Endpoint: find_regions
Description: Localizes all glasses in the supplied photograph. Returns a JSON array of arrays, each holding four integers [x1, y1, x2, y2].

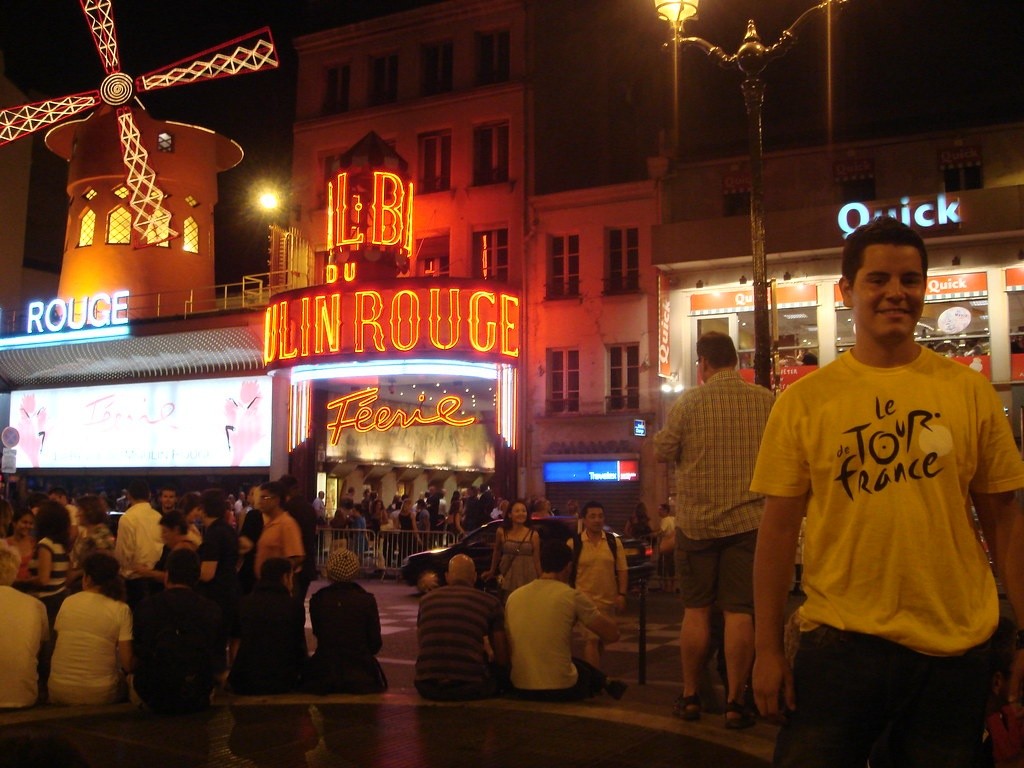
[[260, 495, 276, 501]]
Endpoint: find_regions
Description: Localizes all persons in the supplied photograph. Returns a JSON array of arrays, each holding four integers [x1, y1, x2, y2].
[[567, 502, 628, 668], [625, 502, 677, 592], [504, 542, 630, 700], [1011, 336, 1024, 353], [313, 491, 324, 551], [529, 492, 580, 517], [414, 554, 508, 700], [920, 340, 981, 356], [331, 484, 509, 566], [654, 331, 776, 727], [0, 475, 317, 667], [481, 501, 543, 595], [751, 218, 1024, 768], [800, 343, 817, 364], [0, 549, 388, 711]]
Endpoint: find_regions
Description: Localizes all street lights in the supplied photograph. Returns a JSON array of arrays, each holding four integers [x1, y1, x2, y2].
[[653, 1, 847, 391]]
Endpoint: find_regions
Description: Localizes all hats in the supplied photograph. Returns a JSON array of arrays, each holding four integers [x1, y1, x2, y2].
[[327, 547, 360, 583]]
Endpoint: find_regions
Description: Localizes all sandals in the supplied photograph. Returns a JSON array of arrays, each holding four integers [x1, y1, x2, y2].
[[672, 693, 701, 720], [725, 700, 756, 729]]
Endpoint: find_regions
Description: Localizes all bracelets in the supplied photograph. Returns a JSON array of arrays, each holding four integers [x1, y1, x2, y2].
[[619, 592, 626, 596]]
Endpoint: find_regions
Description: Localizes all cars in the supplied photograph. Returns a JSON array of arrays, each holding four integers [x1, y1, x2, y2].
[[400, 516, 658, 596]]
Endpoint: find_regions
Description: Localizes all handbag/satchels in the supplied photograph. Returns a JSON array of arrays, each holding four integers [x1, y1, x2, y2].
[[485, 575, 504, 595], [380, 510, 394, 531]]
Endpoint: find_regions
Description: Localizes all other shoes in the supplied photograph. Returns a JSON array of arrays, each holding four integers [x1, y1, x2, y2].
[[606, 680, 629, 700]]
